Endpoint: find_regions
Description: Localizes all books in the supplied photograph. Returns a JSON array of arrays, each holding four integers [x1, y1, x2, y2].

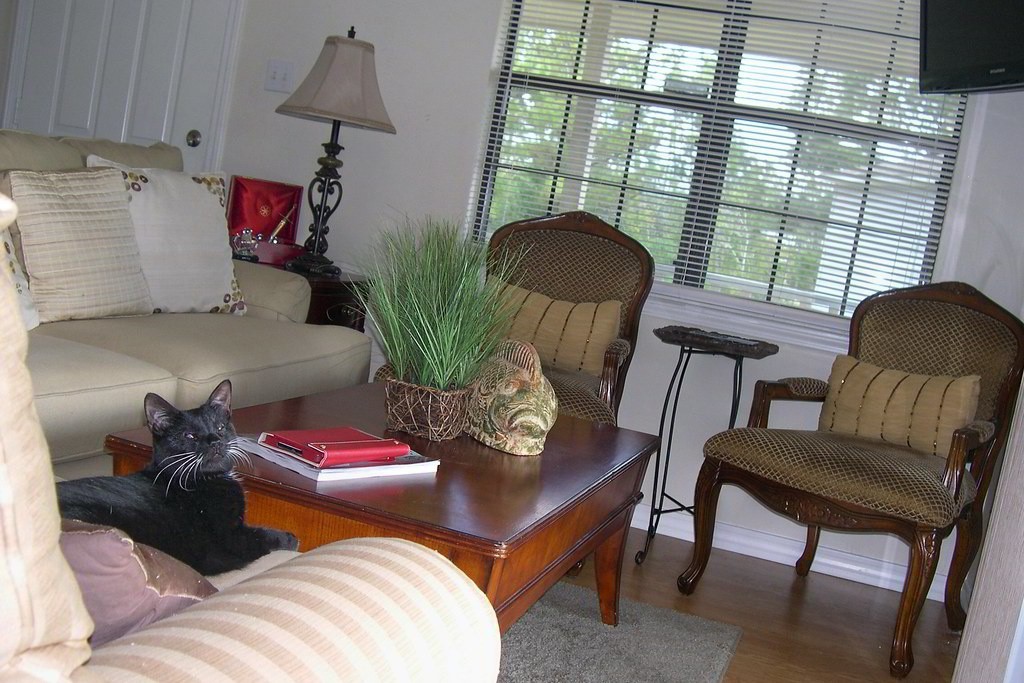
[[235, 426, 441, 482]]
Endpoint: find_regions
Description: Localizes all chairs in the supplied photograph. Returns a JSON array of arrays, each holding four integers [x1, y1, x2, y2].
[[677, 280, 1024, 678], [461, 210, 656, 578]]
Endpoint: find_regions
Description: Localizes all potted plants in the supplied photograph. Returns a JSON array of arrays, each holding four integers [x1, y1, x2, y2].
[[340, 226, 536, 439]]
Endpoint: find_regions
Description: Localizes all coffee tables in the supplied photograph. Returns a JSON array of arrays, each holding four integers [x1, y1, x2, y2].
[[102, 382, 654, 640]]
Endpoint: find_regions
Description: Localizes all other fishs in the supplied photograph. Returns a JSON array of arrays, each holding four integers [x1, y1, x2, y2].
[[464, 337, 559, 455]]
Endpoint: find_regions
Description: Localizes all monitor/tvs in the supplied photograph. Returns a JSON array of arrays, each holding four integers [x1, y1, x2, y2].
[[918, 0, 1024, 95]]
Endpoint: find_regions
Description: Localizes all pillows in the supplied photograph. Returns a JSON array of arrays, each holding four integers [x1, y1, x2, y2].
[[482, 274, 622, 379], [819, 354, 984, 455], [59, 516, 220, 653], [3, 156, 241, 331]]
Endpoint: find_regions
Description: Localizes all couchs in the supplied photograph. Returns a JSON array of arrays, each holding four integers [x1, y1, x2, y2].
[[0, 128, 506, 682]]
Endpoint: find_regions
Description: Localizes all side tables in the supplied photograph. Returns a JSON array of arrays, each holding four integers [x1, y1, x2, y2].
[[255, 257, 373, 334]]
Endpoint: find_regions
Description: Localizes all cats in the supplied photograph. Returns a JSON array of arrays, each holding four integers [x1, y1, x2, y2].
[[53, 378, 299, 576]]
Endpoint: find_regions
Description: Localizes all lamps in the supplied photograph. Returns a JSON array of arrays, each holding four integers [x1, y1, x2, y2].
[[275, 25, 397, 275]]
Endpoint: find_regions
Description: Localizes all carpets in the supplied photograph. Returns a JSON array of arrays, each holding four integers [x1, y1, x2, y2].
[[499, 593, 748, 682]]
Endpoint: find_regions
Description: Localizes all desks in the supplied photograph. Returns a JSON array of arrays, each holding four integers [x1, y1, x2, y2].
[[636, 326, 778, 561]]
